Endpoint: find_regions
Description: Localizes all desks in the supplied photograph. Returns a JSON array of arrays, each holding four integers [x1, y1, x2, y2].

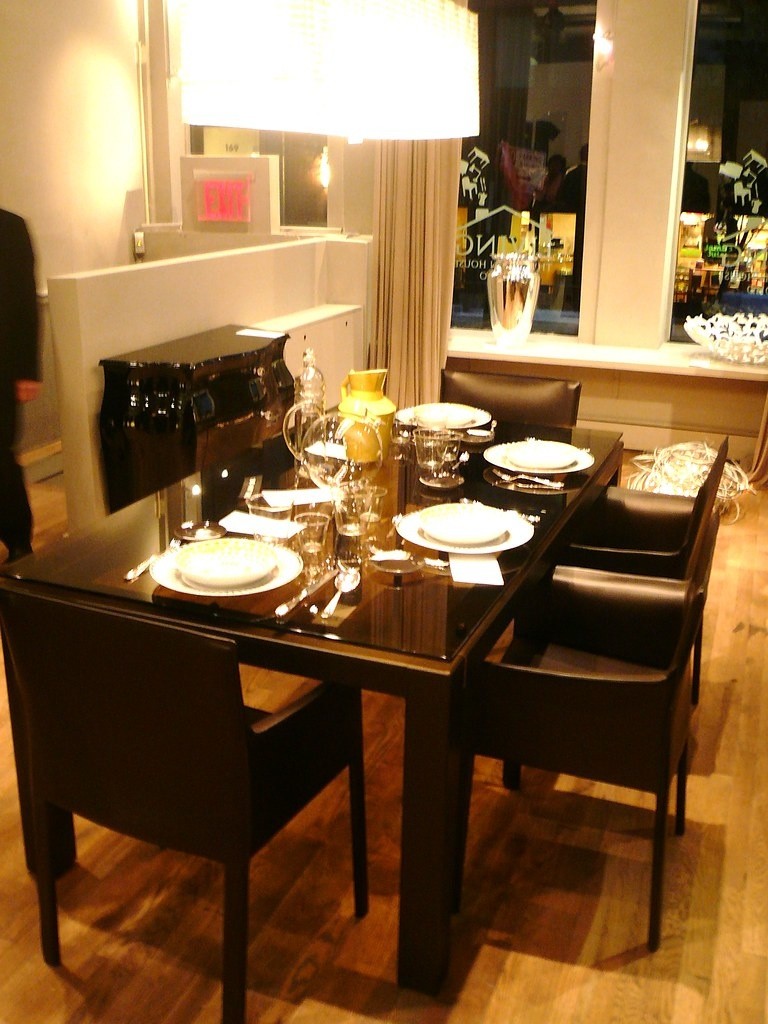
[[100, 324, 294, 515], [0, 404, 624, 997]]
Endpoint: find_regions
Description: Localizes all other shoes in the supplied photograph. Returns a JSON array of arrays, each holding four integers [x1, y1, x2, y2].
[[7, 546, 32, 562]]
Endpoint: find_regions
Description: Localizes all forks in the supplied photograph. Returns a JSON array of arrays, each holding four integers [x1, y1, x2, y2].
[[492, 468, 565, 489], [124, 539, 181, 581]]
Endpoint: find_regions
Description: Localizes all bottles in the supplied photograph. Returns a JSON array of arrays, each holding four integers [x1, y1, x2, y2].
[[293, 349, 326, 453], [487, 251, 540, 348]]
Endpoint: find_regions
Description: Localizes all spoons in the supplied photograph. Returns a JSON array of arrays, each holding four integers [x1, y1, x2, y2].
[[451, 451, 469, 469], [322, 568, 360, 619], [491, 419, 497, 433]]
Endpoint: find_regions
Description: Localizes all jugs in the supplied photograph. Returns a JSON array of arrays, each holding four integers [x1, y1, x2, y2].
[[338, 369, 395, 464]]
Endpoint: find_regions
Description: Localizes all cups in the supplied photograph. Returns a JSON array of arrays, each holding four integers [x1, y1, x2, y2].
[[294, 512, 329, 554], [433, 432, 463, 462], [412, 428, 450, 470], [245, 494, 294, 548], [333, 486, 377, 563], [352, 486, 387, 524]]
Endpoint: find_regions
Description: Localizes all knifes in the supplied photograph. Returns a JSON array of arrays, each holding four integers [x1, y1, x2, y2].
[[275, 569, 340, 617]]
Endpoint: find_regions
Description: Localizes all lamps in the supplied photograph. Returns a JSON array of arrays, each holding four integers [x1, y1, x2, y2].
[[178, 2, 480, 140]]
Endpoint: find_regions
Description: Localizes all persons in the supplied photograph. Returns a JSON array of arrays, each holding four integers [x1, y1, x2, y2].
[[0, 205, 45, 564], [531, 141, 588, 312], [673, 162, 713, 320]]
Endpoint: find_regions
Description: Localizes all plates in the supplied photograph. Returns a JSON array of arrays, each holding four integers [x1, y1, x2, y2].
[[174, 521, 227, 542], [396, 404, 491, 429], [483, 440, 594, 473], [395, 508, 535, 554], [149, 541, 303, 597]]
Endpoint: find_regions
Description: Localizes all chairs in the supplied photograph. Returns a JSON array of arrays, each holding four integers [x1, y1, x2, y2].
[[439, 369, 582, 428], [512, 437, 735, 704], [454, 512, 727, 952], [3, 588, 371, 1022]]
